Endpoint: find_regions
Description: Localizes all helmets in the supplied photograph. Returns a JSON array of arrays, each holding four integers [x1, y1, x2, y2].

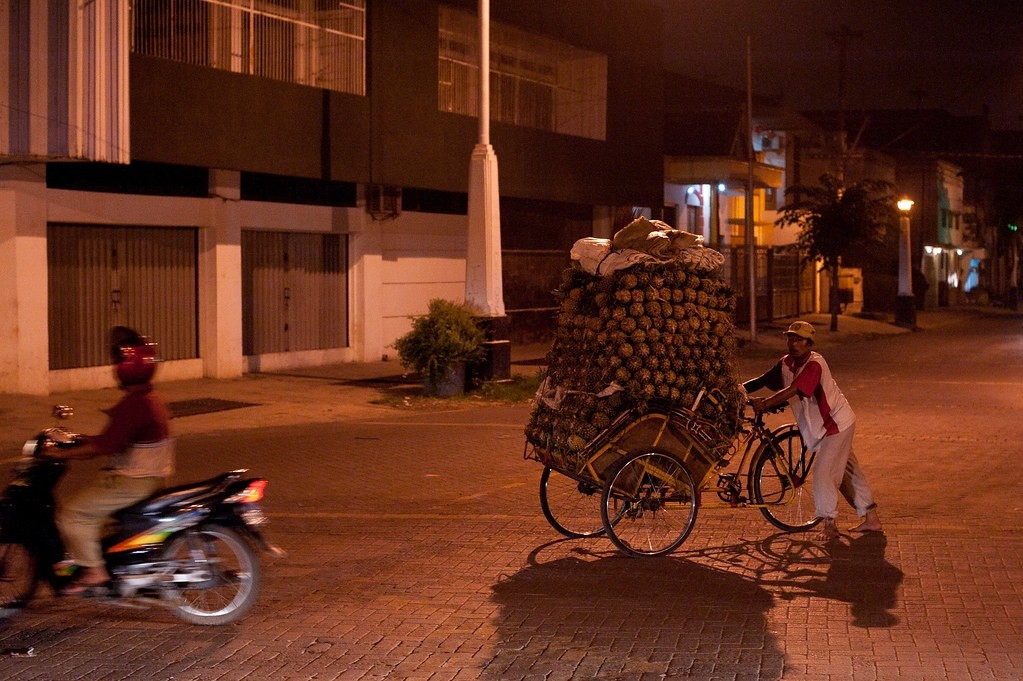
[[113, 345, 158, 387]]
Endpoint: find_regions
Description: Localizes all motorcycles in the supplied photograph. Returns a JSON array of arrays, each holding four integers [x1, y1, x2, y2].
[[0, 405, 287, 627]]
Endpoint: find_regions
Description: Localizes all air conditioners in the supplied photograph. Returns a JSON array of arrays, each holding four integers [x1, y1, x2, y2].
[[368, 184, 402, 216]]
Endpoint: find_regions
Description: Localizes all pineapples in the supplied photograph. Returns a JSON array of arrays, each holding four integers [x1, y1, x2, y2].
[[523, 259, 745, 468]]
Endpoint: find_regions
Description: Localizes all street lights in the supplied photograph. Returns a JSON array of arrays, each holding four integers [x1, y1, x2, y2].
[[895, 197, 918, 326]]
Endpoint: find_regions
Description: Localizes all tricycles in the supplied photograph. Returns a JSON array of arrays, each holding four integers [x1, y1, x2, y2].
[[523, 385, 825, 558]]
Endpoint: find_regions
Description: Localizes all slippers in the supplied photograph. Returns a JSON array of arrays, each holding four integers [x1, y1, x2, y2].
[[60, 580, 112, 594]]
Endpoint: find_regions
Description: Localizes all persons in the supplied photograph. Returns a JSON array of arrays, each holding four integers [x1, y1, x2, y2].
[[39, 325, 177, 594], [742, 320, 883, 541]]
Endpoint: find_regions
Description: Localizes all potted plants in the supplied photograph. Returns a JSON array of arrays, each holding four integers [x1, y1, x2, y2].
[[394, 297, 486, 399]]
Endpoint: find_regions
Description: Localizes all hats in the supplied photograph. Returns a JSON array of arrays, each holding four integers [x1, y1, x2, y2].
[[782, 321, 817, 345]]
